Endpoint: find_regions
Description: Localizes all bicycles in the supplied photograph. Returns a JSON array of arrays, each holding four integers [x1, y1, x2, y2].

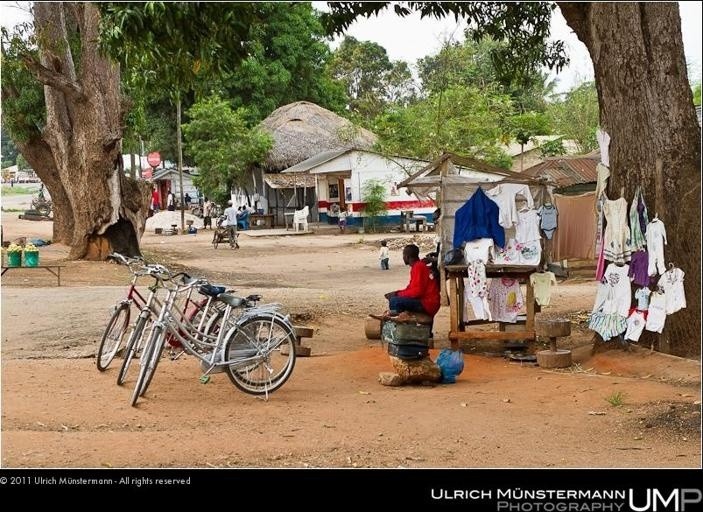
[[97, 251, 296, 406]]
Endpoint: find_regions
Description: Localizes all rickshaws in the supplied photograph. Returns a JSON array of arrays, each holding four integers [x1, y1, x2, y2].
[[212, 227, 240, 249]]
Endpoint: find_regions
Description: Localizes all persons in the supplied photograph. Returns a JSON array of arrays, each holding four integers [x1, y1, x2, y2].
[[10, 179, 13, 187], [330, 202, 353, 228], [384, 244, 442, 317], [167, 191, 174, 211], [38, 189, 43, 198], [238, 202, 254, 219], [152, 188, 159, 214], [172, 194, 179, 210], [202, 197, 212, 230], [379, 240, 390, 269], [185, 193, 192, 210], [221, 201, 239, 249]]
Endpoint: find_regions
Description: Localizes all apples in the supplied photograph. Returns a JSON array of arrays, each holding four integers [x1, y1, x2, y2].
[[7, 240, 40, 252]]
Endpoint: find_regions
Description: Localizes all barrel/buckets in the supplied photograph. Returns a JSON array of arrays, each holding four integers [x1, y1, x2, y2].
[[24, 250, 39, 268], [6, 249, 21, 267]]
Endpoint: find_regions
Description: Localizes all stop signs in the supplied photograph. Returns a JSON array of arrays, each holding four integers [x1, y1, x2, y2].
[[147, 151, 161, 167]]
